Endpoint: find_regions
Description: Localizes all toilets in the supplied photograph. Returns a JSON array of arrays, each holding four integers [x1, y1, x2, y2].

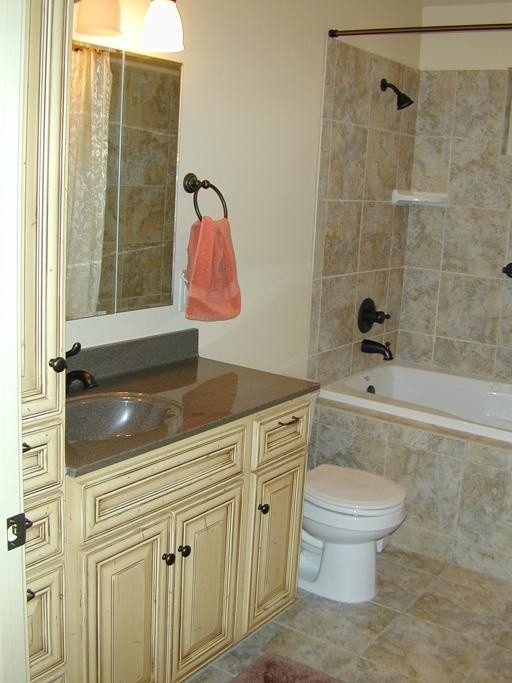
[[296, 462, 408, 602]]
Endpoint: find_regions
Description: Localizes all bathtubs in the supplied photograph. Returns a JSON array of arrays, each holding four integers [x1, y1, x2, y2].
[[318, 360, 512, 443]]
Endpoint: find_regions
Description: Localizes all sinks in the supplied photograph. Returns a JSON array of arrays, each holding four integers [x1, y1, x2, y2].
[[65, 390, 184, 441]]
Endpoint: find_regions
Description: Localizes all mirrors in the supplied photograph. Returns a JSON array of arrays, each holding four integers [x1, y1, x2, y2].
[[66, 39, 183, 321]]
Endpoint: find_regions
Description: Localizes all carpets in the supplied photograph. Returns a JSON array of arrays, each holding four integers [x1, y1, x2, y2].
[[228, 652, 348, 683]]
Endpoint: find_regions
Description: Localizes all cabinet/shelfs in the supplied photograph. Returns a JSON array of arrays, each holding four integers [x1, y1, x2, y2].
[[241, 393, 320, 639], [21, 0, 74, 423], [65, 415, 248, 683], [21, 417, 69, 682]]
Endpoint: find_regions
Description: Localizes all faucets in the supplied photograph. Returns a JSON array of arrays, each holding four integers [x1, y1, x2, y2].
[[360, 338, 393, 360], [66, 370, 98, 398]]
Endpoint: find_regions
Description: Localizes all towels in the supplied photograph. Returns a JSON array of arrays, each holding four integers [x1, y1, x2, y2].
[[180, 216, 242, 321]]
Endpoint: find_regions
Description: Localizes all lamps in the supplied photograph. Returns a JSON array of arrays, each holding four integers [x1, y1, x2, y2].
[[137, 0, 183, 54], [76, 1, 121, 38]]
